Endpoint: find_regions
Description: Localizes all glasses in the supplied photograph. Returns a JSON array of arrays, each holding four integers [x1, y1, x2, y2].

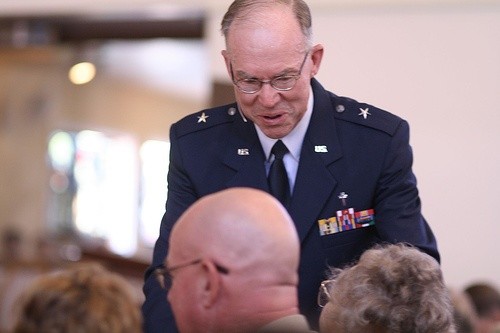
[[230, 51, 308, 94], [317, 280, 334, 307], [151, 258, 228, 290]]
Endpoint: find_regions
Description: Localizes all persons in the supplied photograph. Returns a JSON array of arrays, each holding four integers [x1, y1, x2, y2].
[[317, 242, 457, 333], [155, 186, 315, 333], [9, 264, 141, 333], [141, 0, 441, 333]]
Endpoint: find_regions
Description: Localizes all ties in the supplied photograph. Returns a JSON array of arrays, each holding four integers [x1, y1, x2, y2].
[[267, 140, 290, 207]]
